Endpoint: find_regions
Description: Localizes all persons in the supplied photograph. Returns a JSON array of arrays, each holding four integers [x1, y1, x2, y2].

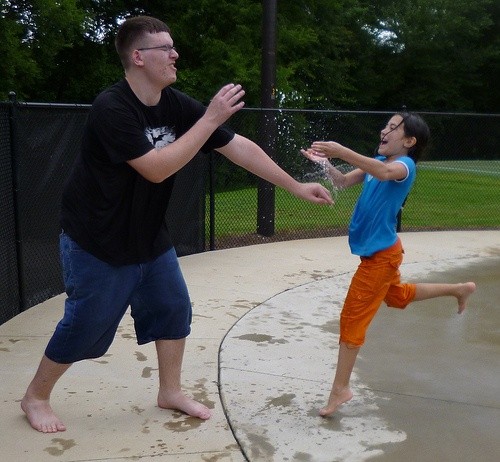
[[20, 16, 335, 433], [300, 110, 476, 415]]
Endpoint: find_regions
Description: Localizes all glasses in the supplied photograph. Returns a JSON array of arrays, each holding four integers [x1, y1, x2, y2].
[[138, 44, 176, 52]]
